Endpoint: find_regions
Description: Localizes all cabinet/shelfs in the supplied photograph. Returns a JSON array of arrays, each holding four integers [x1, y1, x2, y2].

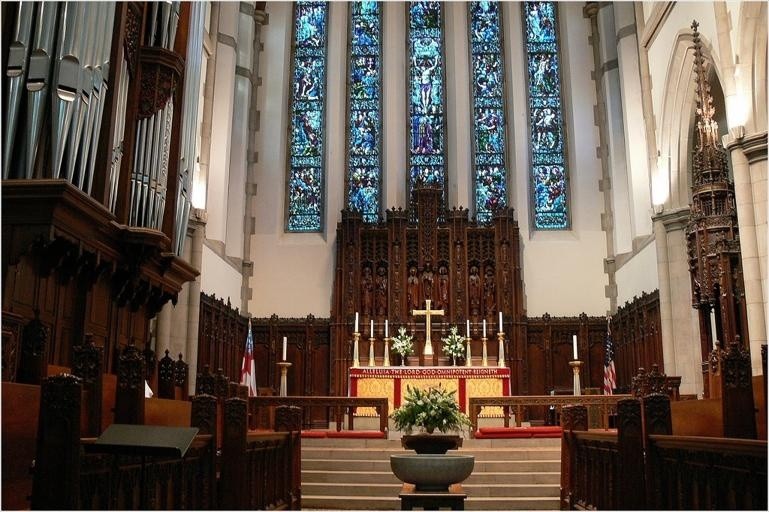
[[2, 311, 302, 511]]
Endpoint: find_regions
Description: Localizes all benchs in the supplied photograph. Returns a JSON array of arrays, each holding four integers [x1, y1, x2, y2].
[[558, 328, 768, 510]]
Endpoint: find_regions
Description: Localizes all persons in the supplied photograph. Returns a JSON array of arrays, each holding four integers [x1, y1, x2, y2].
[[361, 266, 497, 314]]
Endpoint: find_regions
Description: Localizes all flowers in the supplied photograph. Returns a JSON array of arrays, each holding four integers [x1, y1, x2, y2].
[[440, 325, 467, 361], [389, 383, 477, 434], [390, 326, 412, 356]]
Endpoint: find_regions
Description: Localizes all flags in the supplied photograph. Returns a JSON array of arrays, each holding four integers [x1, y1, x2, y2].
[[239, 318, 257, 397], [603, 318, 618, 395]]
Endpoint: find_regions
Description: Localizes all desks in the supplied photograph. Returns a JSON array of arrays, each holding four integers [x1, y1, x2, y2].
[[248, 394, 389, 439], [347, 365, 512, 418], [469, 393, 697, 438]]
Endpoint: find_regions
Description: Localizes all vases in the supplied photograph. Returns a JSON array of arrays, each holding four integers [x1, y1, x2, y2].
[[452, 357, 458, 367], [400, 357, 405, 367], [401, 435, 463, 456]]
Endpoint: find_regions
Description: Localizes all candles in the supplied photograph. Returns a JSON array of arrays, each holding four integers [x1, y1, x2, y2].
[[467, 319, 470, 337], [499, 311, 503, 332], [483, 319, 486, 337], [385, 320, 389, 337], [355, 312, 358, 332], [371, 320, 374, 337]]
